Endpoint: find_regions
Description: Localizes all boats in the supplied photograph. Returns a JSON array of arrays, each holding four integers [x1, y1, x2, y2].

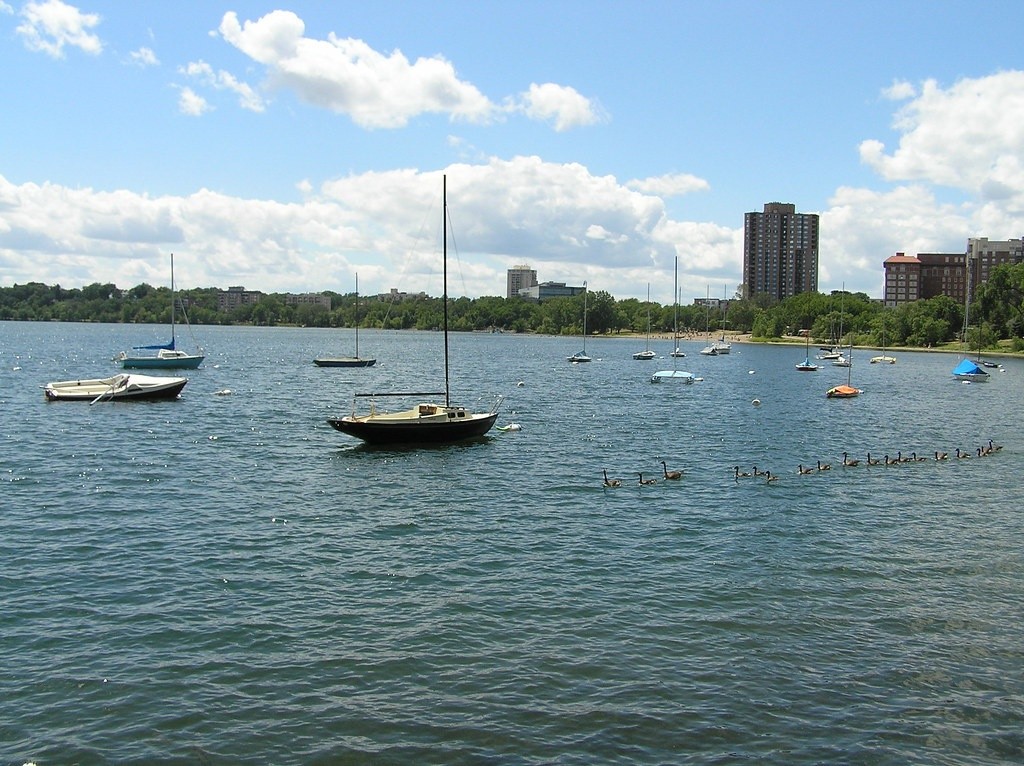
[[566, 281, 593, 362], [325, 170, 499, 445], [650, 256, 695, 385], [951, 359, 990, 383], [310, 271, 377, 367], [669, 284, 686, 358], [39, 372, 189, 403], [120, 252, 206, 369], [870, 285, 897, 365], [815, 282, 855, 367], [701, 285, 718, 355], [629, 281, 658, 360], [714, 285, 732, 354], [795, 338, 818, 370], [826, 334, 864, 396]]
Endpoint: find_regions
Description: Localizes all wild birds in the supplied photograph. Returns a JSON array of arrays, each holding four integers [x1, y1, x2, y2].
[[867, 451, 928, 466], [797, 464, 815, 476], [817, 460, 833, 472], [842, 452, 860, 467], [734, 465, 780, 486], [976, 438, 1003, 458], [935, 448, 971, 461], [601, 460, 684, 489]]
